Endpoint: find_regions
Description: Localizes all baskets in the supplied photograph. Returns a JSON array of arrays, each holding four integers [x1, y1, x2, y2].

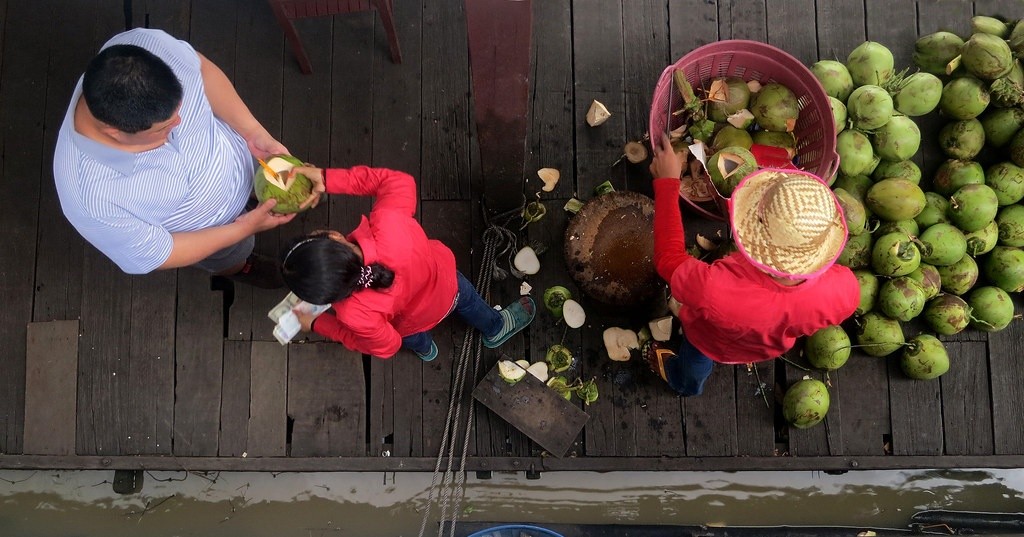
[[649, 40, 840, 223]]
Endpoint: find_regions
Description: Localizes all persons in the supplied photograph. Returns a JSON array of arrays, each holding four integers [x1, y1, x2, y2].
[[638, 130, 859, 398], [53, 26, 297, 288], [292, 164, 536, 361]]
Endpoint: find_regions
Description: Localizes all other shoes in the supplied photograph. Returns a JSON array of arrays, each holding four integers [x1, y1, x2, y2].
[[236, 242, 277, 278]]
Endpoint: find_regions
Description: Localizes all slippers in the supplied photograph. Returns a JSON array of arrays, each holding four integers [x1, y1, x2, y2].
[[482, 296, 536, 348], [642, 340, 676, 382], [412, 339, 439, 362]]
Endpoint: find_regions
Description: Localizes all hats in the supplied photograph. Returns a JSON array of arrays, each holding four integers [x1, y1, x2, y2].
[[730, 168, 847, 280]]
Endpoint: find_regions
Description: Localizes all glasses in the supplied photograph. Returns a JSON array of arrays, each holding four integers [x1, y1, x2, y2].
[[283, 236, 324, 275]]
